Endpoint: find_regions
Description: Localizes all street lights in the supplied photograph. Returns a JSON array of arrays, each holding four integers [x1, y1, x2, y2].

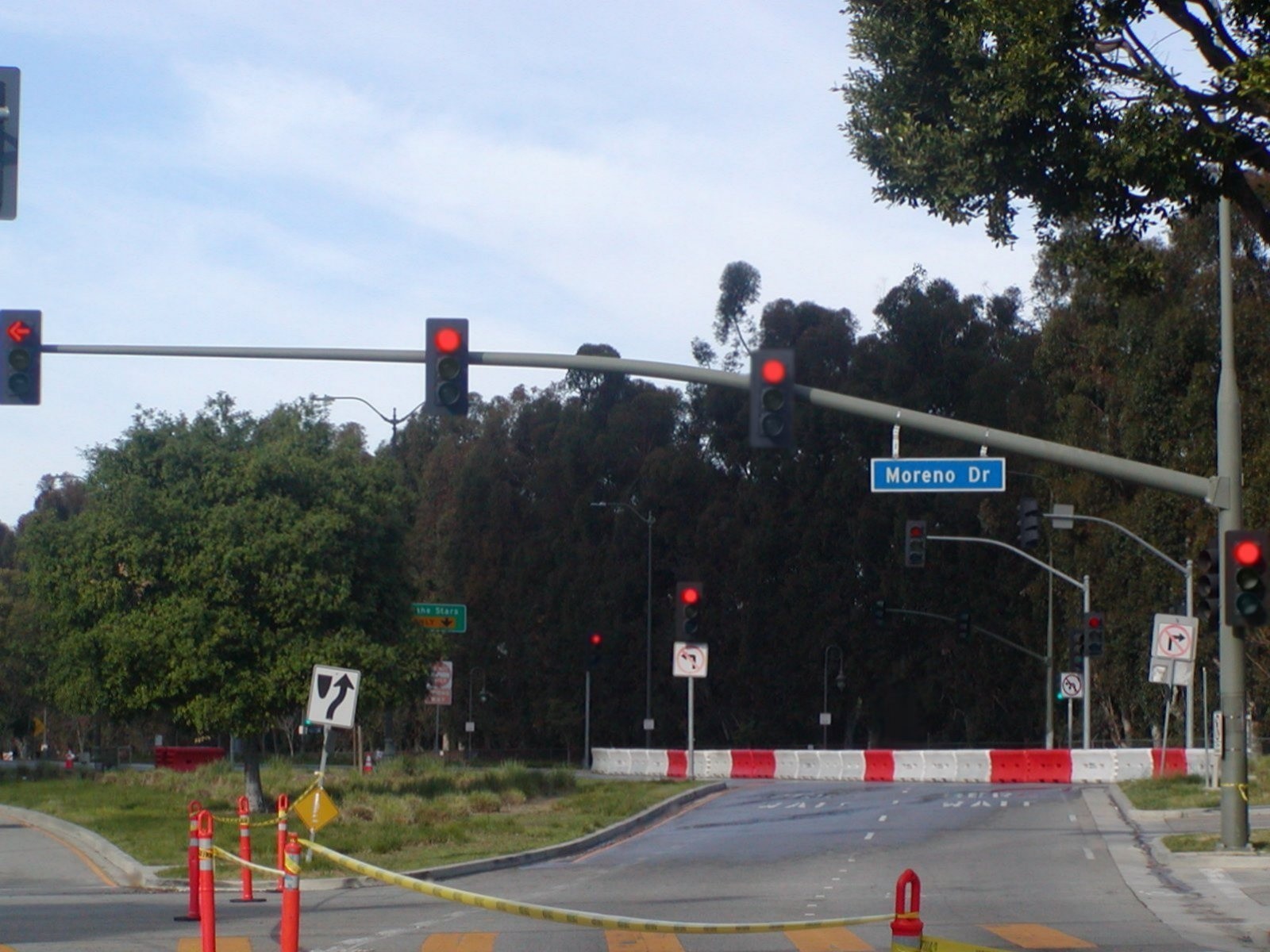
[[824, 644, 848, 748], [588, 491, 654, 747], [467, 667, 489, 757]]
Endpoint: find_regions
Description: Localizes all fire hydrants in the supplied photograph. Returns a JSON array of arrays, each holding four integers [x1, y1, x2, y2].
[[66, 749, 76, 770]]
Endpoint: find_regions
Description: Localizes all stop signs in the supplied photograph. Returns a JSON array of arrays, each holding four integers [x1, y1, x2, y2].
[[428, 660, 453, 686]]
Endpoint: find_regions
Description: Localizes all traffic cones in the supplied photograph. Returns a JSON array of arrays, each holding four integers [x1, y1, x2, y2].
[[364, 753, 373, 773]]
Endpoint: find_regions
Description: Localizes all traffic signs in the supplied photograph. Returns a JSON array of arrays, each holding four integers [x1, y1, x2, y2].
[[411, 604, 466, 633]]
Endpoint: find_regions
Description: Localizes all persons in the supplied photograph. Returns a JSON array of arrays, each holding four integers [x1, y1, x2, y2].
[[64, 743, 75, 769]]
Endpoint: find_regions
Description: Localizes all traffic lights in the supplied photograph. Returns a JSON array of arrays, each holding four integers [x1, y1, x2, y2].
[[1085, 614, 1106, 657], [954, 610, 972, 646], [752, 352, 797, 450], [588, 630, 612, 666], [426, 318, 468, 415], [1223, 528, 1270, 629], [876, 600, 886, 632], [673, 583, 706, 642], [0, 310, 41, 405], [1198, 541, 1221, 631], [1070, 630, 1084, 670], [1053, 671, 1066, 703], [905, 522, 929, 566], [1017, 500, 1042, 549]]
